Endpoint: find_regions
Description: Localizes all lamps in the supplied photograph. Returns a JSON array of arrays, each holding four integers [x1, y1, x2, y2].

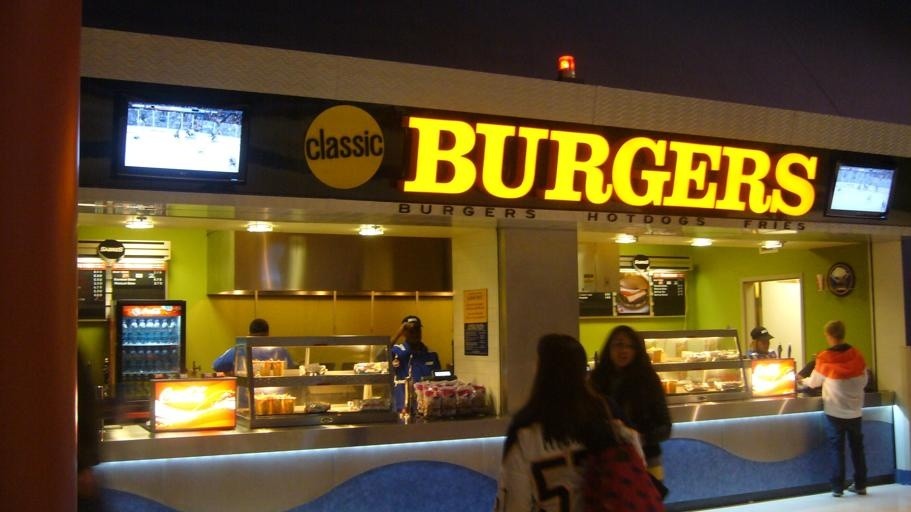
[[124, 216, 156, 230], [357, 223, 385, 235], [613, 233, 638, 244], [690, 239, 714, 248], [761, 239, 784, 248], [557, 55, 576, 82], [245, 221, 275, 233]]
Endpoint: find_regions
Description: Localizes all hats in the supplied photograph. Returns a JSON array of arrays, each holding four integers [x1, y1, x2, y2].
[[402, 315, 424, 328], [750, 326, 775, 340]]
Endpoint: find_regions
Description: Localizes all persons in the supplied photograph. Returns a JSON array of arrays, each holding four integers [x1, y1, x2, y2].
[[375, 316, 441, 412], [590, 325, 672, 481], [747, 326, 777, 356], [213, 318, 298, 409], [796, 320, 868, 497], [494, 333, 646, 512]]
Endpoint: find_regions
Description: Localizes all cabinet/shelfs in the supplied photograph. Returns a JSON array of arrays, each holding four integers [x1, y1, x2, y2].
[[637, 330, 751, 405], [231, 335, 399, 430]]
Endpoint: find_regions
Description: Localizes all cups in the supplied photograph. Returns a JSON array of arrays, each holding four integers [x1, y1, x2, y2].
[[254, 397, 267, 414], [670, 381, 678, 392], [252, 361, 258, 374], [284, 398, 294, 413], [273, 361, 285, 376], [269, 399, 282, 413], [262, 362, 271, 377], [654, 350, 661, 361]]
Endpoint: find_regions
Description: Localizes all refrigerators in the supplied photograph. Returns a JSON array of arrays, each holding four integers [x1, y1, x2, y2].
[[116, 298, 187, 410]]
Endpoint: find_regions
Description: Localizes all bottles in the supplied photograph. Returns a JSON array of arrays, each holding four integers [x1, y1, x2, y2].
[[162, 350, 171, 370], [137, 350, 146, 373], [146, 350, 154, 371], [122, 375, 150, 400], [154, 349, 162, 371], [146, 319, 154, 344], [128, 350, 137, 371], [139, 319, 146, 344], [171, 350, 178, 369], [153, 319, 161, 343], [122, 319, 128, 344], [161, 319, 169, 343], [129, 318, 138, 344], [169, 319, 178, 343]]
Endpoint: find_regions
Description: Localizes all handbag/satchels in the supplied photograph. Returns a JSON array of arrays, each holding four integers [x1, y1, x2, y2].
[[580, 439, 665, 512]]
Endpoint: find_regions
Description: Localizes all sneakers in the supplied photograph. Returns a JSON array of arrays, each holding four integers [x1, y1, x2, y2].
[[832, 490, 841, 497], [848, 482, 866, 495]]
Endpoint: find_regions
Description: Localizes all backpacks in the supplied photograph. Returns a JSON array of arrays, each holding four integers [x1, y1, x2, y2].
[[580, 412, 670, 512]]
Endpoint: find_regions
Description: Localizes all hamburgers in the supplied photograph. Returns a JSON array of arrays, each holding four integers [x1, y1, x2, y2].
[[617, 276, 650, 309]]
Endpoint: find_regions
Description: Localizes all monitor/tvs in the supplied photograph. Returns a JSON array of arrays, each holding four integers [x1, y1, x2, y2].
[[822, 159, 900, 221], [109, 96, 251, 184]]
